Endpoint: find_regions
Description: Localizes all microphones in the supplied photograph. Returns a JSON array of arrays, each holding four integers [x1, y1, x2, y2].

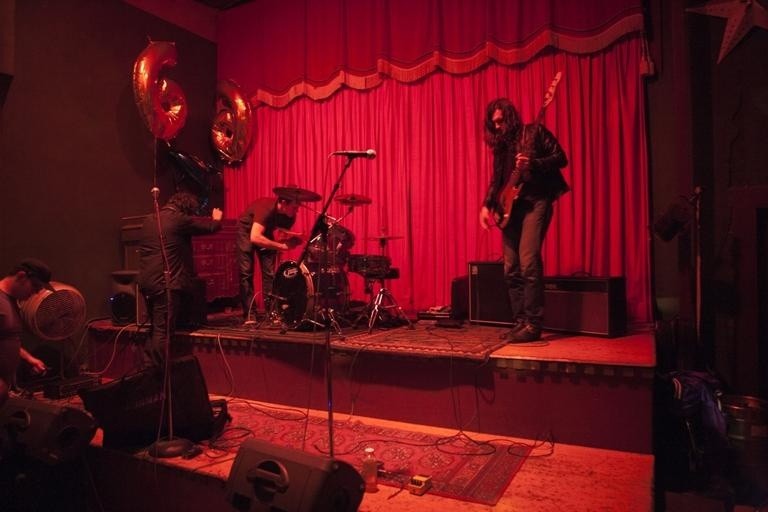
[[333, 149, 376, 159], [151, 186, 160, 204]]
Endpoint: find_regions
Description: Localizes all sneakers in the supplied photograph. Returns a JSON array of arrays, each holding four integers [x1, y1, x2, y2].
[[508, 324, 542, 342], [500, 323, 524, 339], [245, 314, 257, 325], [269, 320, 280, 327]]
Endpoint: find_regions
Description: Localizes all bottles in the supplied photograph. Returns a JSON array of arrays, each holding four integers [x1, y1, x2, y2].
[[362, 445, 379, 495]]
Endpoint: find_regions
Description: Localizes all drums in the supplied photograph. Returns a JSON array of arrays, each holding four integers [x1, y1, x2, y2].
[[17, 279, 87, 341], [311, 226, 353, 306], [273, 261, 315, 326], [348, 254, 390, 274]]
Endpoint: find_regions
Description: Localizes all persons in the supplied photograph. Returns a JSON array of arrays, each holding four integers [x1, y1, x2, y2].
[[237, 192, 305, 327], [477, 98, 569, 341], [139, 191, 224, 425], [0, 257, 50, 410]]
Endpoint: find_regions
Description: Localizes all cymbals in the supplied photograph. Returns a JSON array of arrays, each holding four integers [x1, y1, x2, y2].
[[333, 193, 372, 204], [361, 234, 402, 241], [273, 187, 319, 202]]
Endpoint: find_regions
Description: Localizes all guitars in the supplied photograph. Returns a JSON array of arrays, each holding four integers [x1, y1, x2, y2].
[[486, 71, 562, 229]]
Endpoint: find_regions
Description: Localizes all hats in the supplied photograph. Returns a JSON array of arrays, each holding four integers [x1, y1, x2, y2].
[[16, 258, 54, 294]]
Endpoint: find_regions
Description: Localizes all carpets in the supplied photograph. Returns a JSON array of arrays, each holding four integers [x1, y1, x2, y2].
[[140, 311, 374, 347], [198, 400, 534, 508], [328, 316, 513, 362]]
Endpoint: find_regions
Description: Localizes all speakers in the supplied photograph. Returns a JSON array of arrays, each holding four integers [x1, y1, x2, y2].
[[540, 278, 628, 336], [468, 261, 515, 325], [221, 437, 365, 512], [108, 270, 147, 325], [83, 359, 212, 452]]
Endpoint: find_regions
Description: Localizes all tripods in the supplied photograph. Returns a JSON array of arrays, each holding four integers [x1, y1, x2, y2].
[[355, 254, 414, 332]]
